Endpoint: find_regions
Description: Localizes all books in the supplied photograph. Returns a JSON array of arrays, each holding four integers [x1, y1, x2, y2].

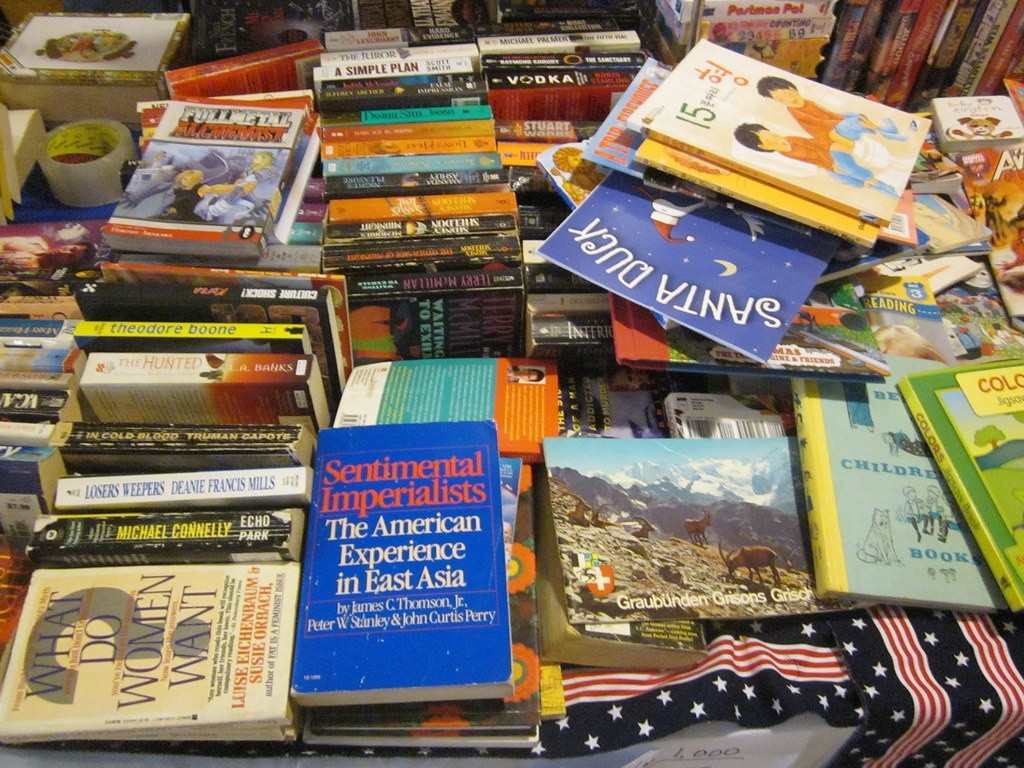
[[164, 39, 325, 110], [103, 96, 314, 254], [627, 39, 933, 227], [291, 420, 516, 706], [25, 509, 303, 562], [0, 0, 1024, 748], [80, 352, 330, 432], [334, 356, 560, 464]]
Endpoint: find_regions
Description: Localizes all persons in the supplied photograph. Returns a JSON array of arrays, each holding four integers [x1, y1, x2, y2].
[[0, 238, 95, 271], [506, 365, 544, 383]]
